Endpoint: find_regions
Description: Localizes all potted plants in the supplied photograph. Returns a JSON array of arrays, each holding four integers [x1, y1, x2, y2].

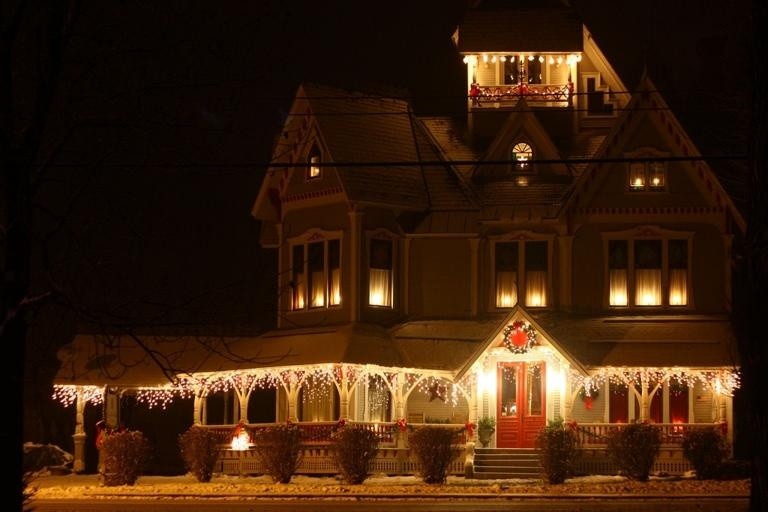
[[478, 416, 496, 447]]
